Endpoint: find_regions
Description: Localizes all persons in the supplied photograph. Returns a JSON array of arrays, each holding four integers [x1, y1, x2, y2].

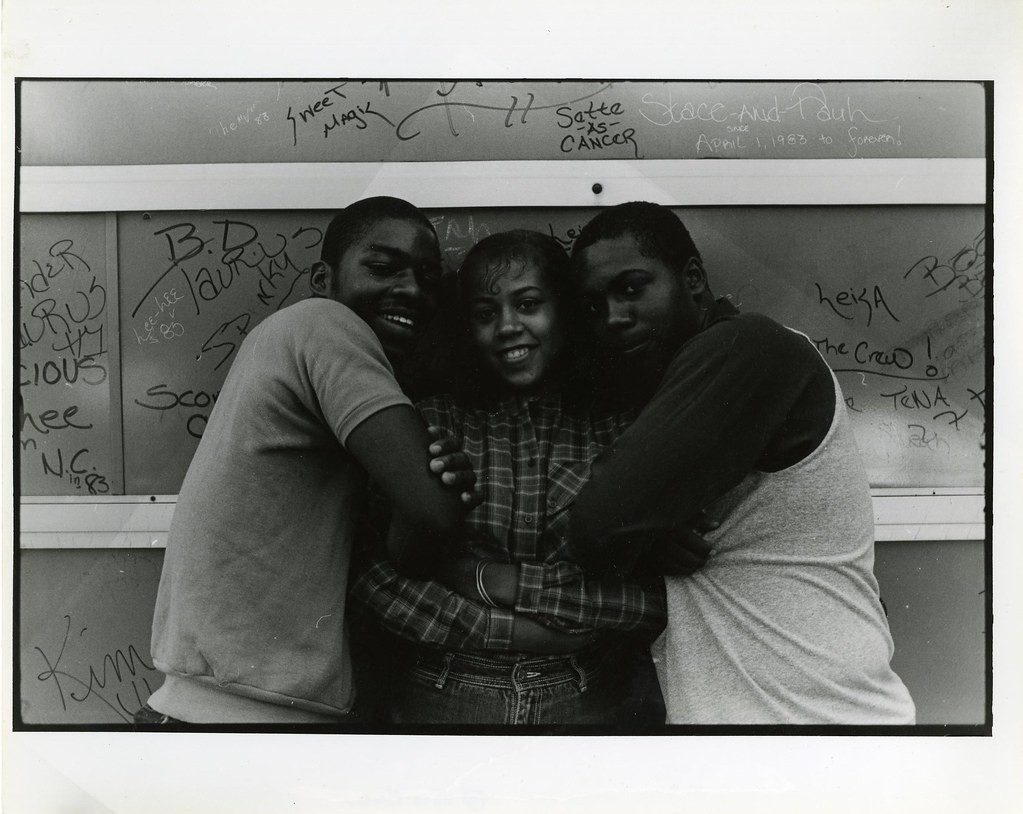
[[568, 201, 917, 724], [132, 198, 484, 722], [347, 228, 667, 724]]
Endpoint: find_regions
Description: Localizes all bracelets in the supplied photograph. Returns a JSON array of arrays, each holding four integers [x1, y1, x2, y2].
[[476, 559, 498, 607]]
